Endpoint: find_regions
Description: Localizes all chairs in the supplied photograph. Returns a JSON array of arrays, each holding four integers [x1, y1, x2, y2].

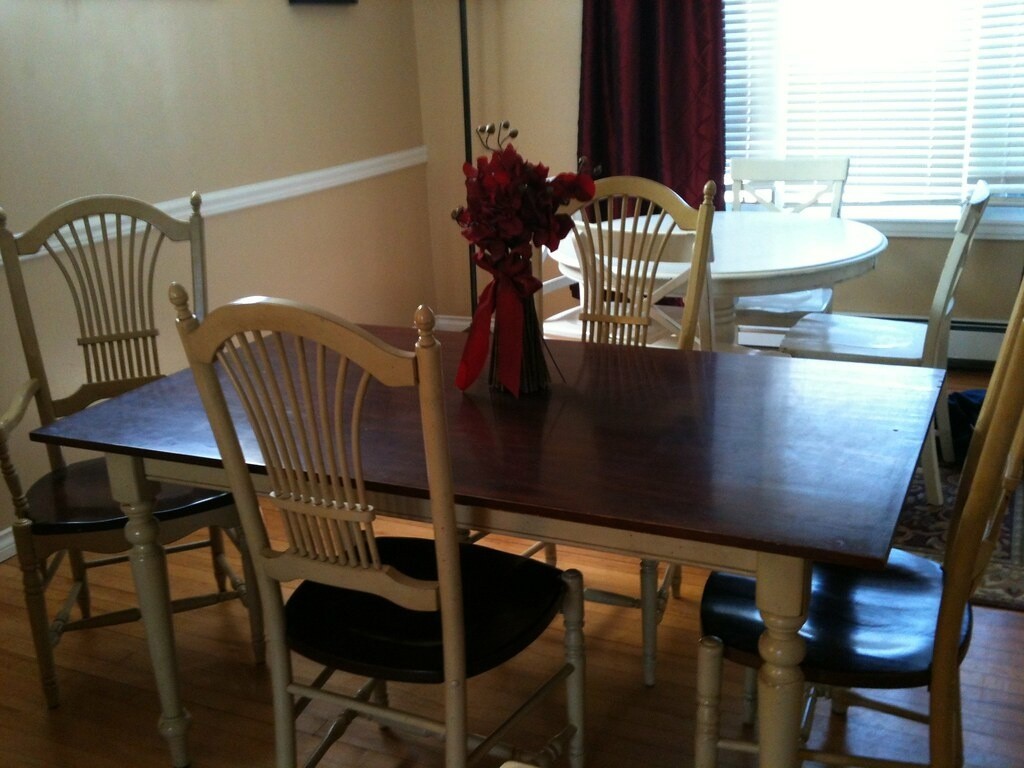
[[693, 276, 1024, 768], [532, 176, 683, 346], [169, 281, 585, 768], [570, 221, 791, 358], [0, 190, 266, 709], [729, 157, 850, 334], [777, 177, 991, 507], [529, 174, 718, 352]]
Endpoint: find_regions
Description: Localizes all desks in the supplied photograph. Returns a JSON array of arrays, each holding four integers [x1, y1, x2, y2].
[[27, 321, 946, 767], [546, 212, 888, 345]]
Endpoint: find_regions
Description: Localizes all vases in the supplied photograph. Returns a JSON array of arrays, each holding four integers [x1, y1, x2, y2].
[[488, 294, 554, 395]]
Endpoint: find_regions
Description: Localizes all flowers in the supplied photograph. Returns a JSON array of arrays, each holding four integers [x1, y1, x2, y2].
[[451, 119, 603, 298]]
